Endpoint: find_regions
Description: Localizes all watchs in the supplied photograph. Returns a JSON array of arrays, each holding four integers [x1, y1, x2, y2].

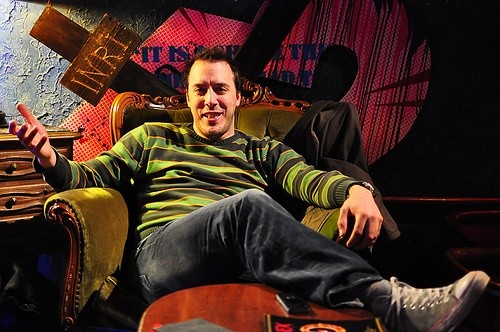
[[360, 181, 377, 194]]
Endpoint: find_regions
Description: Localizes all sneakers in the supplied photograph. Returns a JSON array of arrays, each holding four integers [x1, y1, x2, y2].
[[384, 270, 491, 332]]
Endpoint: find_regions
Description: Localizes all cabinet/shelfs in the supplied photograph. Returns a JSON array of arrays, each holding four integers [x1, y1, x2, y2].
[[0, 127, 82, 226]]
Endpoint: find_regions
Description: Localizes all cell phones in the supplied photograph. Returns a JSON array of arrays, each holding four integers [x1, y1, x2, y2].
[[276, 293, 311, 313]]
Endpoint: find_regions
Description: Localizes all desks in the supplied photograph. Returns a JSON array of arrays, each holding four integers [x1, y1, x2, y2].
[[138, 283, 388, 332]]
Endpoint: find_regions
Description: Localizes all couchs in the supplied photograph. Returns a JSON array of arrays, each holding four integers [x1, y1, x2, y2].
[[43, 78, 401, 332]]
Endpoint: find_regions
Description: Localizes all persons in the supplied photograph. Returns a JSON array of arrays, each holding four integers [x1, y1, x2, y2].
[[7, 49, 492, 332]]
